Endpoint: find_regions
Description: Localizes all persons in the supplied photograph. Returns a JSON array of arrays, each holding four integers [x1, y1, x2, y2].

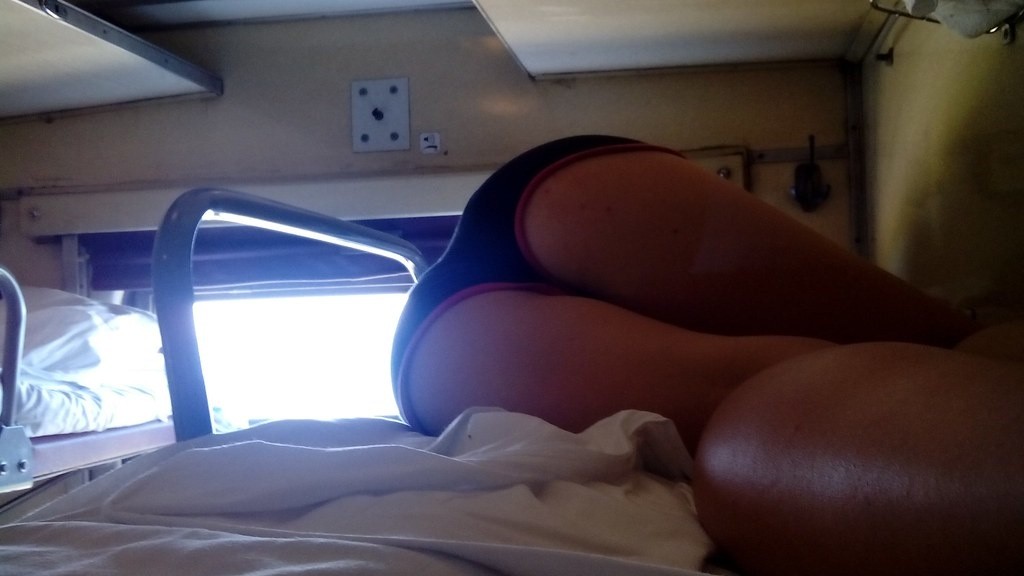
[[391, 135, 1024, 576]]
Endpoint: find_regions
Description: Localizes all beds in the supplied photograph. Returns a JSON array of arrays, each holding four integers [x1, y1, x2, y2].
[[0, 263, 178, 492], [0, 185, 712, 576]]
